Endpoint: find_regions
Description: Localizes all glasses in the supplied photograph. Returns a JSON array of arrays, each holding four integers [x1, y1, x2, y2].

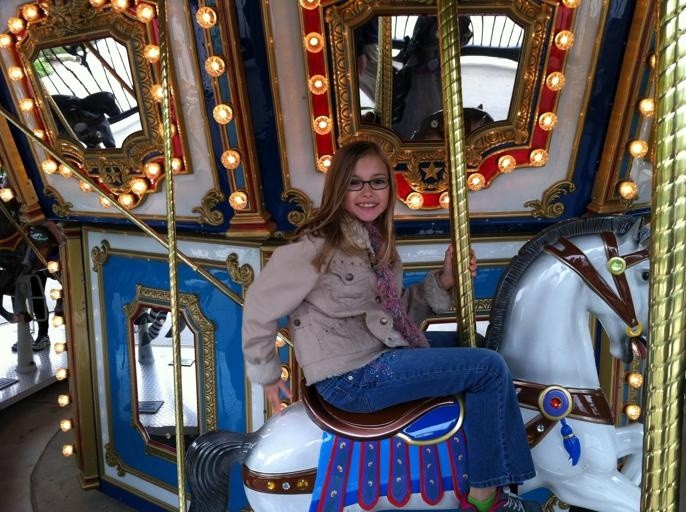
[[347, 178, 389, 191]]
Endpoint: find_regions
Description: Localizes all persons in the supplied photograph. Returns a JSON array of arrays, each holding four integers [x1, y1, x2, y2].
[[352, 16, 401, 107], [9, 253, 53, 349], [240, 138, 543, 512], [392, 11, 472, 141]]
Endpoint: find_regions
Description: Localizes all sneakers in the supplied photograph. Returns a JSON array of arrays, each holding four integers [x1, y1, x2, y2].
[[13, 335, 50, 350], [459, 487, 543, 512]]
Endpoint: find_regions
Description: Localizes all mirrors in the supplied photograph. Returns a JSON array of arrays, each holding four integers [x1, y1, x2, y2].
[[120, 281, 221, 470], [582, 2, 652, 221], [0, 1, 195, 215], [292, 0, 577, 215], [420, 313, 497, 352]]
